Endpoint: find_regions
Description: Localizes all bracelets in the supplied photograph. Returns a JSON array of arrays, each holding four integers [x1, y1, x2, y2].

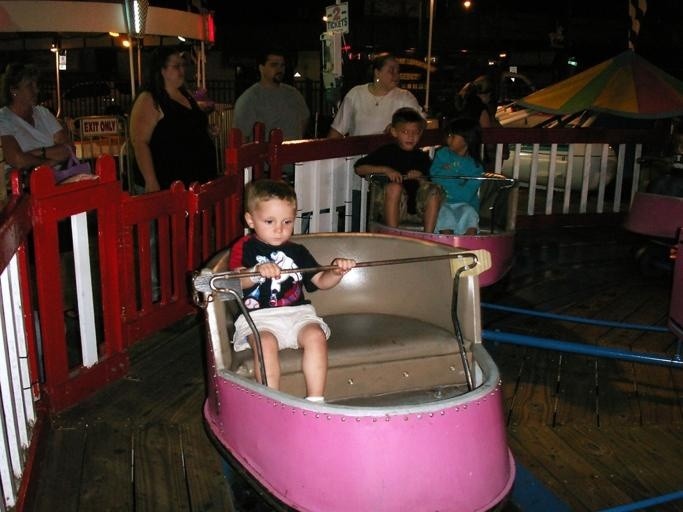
[[40, 146, 48, 159]]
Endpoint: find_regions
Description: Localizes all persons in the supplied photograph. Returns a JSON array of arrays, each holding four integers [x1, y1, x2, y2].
[[649, 126, 683, 195], [0, 63, 77, 170], [445, 71, 510, 171], [355, 105, 483, 235], [225, 176, 356, 406]]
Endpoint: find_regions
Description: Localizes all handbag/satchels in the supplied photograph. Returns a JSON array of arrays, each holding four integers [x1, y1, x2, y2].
[[54, 144, 91, 182]]
